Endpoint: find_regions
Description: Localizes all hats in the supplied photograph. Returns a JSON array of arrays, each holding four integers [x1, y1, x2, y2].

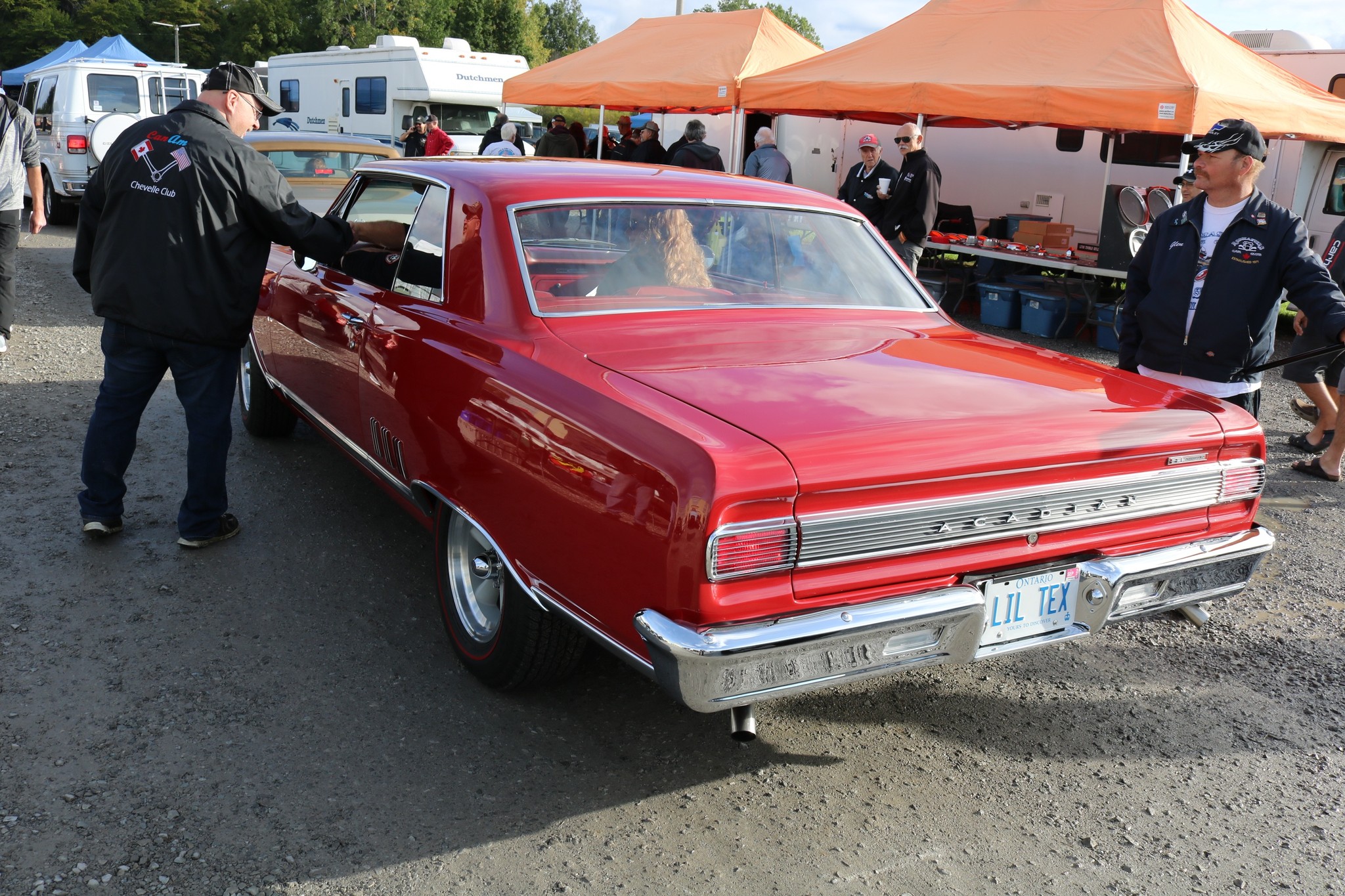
[[636, 121, 660, 132], [423, 114, 437, 123], [201, 61, 286, 117], [415, 115, 426, 124], [571, 122, 585, 136], [597, 126, 609, 137], [616, 115, 632, 125], [1173, 166, 1196, 184], [551, 114, 566, 123], [1181, 118, 1267, 163], [462, 201, 483, 222], [857, 133, 882, 150], [547, 120, 552, 129]]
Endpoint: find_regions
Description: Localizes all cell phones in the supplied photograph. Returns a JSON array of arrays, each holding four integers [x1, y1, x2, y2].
[[413, 127, 416, 131]]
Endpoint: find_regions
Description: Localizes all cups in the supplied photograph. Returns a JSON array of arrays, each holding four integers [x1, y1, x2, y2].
[[879, 178, 891, 195]]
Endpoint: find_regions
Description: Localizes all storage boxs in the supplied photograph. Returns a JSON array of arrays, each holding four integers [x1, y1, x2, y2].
[[916, 266, 963, 314], [976, 281, 1124, 352], [1077, 242, 1099, 253], [1006, 213, 1074, 248]]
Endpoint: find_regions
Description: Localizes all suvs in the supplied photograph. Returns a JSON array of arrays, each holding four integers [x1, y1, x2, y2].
[[18, 57, 207, 225]]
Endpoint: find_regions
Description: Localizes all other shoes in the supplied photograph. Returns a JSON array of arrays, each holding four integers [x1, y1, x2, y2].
[[0, 335, 7, 353], [1290, 398, 1319, 425]]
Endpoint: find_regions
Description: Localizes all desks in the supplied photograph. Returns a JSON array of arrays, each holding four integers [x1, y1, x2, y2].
[[925, 240, 1127, 340]]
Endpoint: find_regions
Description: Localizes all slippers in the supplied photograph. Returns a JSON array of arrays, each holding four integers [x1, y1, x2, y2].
[[1292, 457, 1342, 481], [1288, 432, 1325, 452]]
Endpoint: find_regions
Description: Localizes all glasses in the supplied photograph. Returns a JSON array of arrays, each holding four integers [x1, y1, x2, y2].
[[641, 130, 649, 134], [414, 122, 425, 126], [466, 215, 479, 220], [240, 95, 263, 121], [894, 134, 918, 144], [627, 219, 643, 230], [1176, 182, 1195, 189]]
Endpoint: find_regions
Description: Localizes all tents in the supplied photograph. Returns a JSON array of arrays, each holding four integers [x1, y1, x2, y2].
[[502, 8, 827, 243], [732, 0, 1345, 177], [60, 34, 162, 66], [1, 40, 89, 90]]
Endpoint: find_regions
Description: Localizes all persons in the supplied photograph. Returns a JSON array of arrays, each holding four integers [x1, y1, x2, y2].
[[400, 116, 427, 157], [1117, 118, 1345, 420], [398, 201, 485, 322], [477, 113, 526, 156], [0, 74, 47, 353], [595, 204, 714, 296], [1280, 219, 1345, 480], [876, 122, 942, 278], [72, 62, 407, 547], [533, 115, 589, 239], [837, 134, 899, 226], [670, 119, 726, 173], [666, 132, 688, 165], [1173, 165, 1204, 203], [744, 127, 794, 186], [481, 122, 523, 156], [424, 115, 454, 156], [584, 116, 667, 243]]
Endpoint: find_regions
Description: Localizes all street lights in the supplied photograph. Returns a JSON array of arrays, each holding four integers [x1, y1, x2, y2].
[[153, 21, 201, 62]]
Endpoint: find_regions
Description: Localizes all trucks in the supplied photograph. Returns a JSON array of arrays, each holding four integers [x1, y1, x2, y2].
[[662, 32, 1343, 293], [268, 35, 537, 175]]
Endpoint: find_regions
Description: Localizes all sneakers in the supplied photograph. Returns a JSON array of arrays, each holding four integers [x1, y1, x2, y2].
[[82, 519, 124, 535], [177, 513, 241, 548]]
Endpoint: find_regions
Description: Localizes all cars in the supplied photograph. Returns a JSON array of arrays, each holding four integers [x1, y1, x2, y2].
[[239, 156, 1277, 750], [241, 133, 417, 206]]
